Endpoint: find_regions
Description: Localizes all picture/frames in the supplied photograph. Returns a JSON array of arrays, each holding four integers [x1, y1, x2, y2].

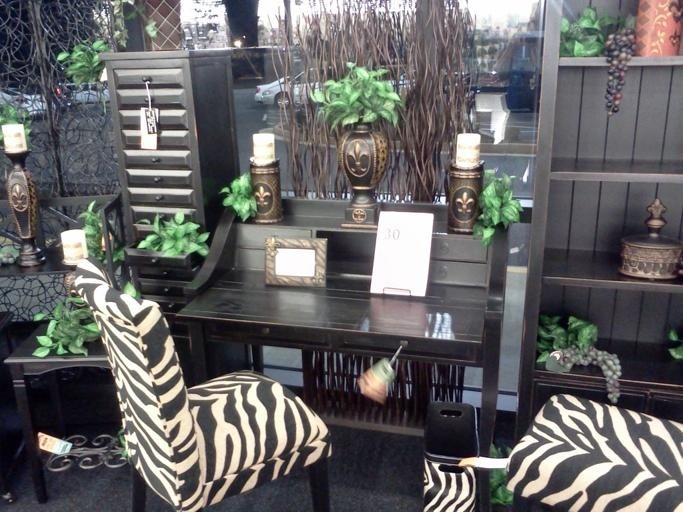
[[265, 237, 327, 288]]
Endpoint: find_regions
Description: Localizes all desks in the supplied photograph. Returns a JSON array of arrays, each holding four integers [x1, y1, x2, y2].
[[172, 199, 505, 511], [459, 85, 511, 145], [0, 321, 146, 511]]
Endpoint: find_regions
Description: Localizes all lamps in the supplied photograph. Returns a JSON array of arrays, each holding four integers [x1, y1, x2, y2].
[[355, 341, 407, 406]]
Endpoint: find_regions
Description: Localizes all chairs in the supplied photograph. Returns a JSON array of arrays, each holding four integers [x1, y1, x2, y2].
[[71, 256, 333, 512], [506, 394, 683, 512]]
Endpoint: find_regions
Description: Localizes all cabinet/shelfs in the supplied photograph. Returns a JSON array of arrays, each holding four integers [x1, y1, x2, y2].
[[98, 48, 235, 383], [1, 193, 139, 506], [124, 236, 200, 386], [517, 0, 683, 425]]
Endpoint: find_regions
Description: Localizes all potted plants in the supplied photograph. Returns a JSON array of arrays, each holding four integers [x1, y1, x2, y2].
[[307, 63, 406, 231]]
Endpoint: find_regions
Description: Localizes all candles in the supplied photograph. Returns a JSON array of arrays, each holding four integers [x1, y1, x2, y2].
[[1, 123, 27, 153], [253, 133, 276, 166], [456, 132, 481, 170]]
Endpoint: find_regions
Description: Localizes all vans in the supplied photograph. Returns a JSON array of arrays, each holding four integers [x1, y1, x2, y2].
[[227, 46, 287, 82]]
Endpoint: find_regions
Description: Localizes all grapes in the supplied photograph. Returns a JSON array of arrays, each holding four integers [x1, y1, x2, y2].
[[560, 345, 623, 403], [605, 29, 637, 116]]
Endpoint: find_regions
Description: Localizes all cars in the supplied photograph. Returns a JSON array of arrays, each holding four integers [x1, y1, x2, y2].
[[254, 76, 293, 109], [262, 67, 343, 110], [380, 65, 511, 114], [0, 82, 110, 118]]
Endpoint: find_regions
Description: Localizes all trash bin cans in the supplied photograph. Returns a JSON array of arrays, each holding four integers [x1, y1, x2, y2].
[[421, 401, 480, 512]]
